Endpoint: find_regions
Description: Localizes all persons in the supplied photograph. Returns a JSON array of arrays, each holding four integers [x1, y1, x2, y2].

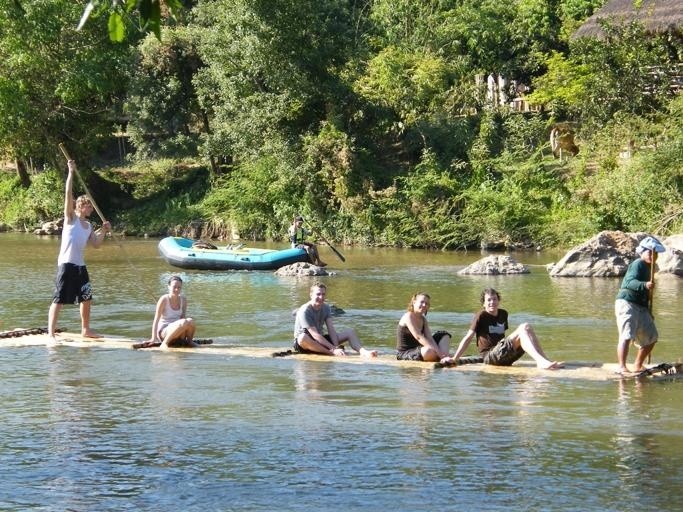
[[285, 218, 328, 268], [293, 282, 380, 358], [139, 277, 202, 350], [615, 237, 664, 374], [441, 287, 565, 371], [398, 292, 452, 363], [47, 159, 108, 340]]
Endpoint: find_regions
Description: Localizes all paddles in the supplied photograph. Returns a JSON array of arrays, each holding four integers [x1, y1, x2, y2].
[[304, 218, 345, 262]]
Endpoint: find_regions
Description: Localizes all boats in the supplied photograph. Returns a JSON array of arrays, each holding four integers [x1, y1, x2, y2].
[[1, 325, 683, 382], [157, 235, 319, 271]]
